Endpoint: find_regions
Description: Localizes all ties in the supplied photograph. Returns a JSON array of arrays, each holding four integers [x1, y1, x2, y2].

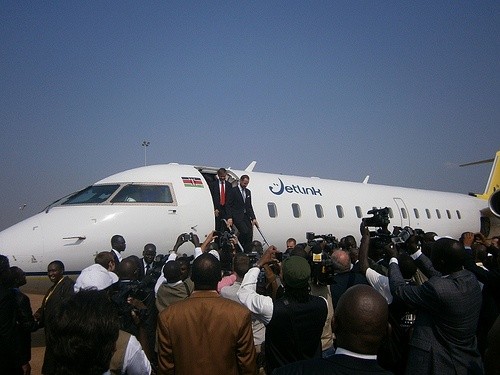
[[221, 181, 225, 206], [242, 188, 246, 214], [145, 265, 150, 275]]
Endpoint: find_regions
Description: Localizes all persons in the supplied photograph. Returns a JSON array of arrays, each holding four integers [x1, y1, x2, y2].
[[0, 232, 500, 375], [211, 168, 232, 235], [225, 174, 260, 253]]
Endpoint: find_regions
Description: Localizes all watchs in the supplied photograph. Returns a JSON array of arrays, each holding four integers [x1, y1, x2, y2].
[[253, 263, 261, 268]]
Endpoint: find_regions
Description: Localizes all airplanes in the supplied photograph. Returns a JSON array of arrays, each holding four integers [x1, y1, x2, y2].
[[0, 151, 500, 292]]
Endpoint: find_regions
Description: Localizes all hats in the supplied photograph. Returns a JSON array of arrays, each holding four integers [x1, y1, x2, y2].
[[74, 264, 119, 293], [283, 256, 311, 287], [177, 240, 196, 264]]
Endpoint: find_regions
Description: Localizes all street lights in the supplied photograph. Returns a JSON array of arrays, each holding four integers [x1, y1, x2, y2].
[[142, 142, 151, 167]]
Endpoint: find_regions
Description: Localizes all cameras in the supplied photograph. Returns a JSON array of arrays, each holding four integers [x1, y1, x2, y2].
[[275, 252, 282, 263], [391, 227, 414, 243], [213, 231, 222, 238]]
